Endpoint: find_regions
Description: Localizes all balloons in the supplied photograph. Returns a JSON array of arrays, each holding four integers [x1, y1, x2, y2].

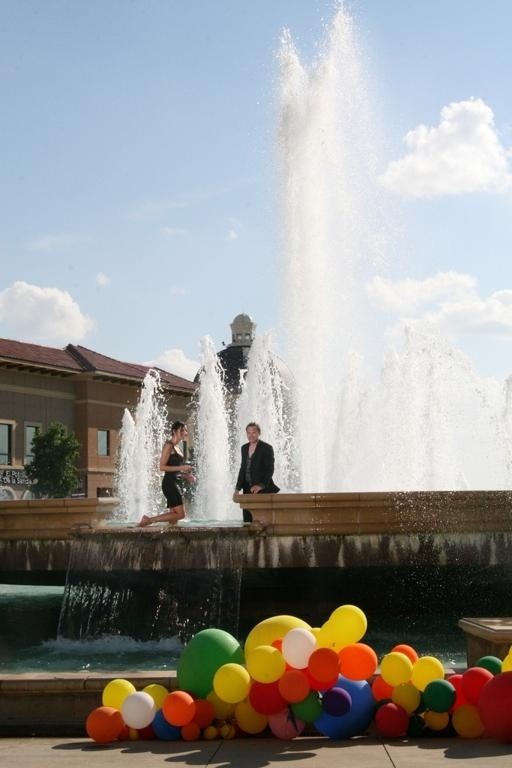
[[87, 605, 512, 741]]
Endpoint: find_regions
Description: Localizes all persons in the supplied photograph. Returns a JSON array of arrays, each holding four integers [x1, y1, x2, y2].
[[138, 421, 192, 527], [233, 422, 280, 523]]
